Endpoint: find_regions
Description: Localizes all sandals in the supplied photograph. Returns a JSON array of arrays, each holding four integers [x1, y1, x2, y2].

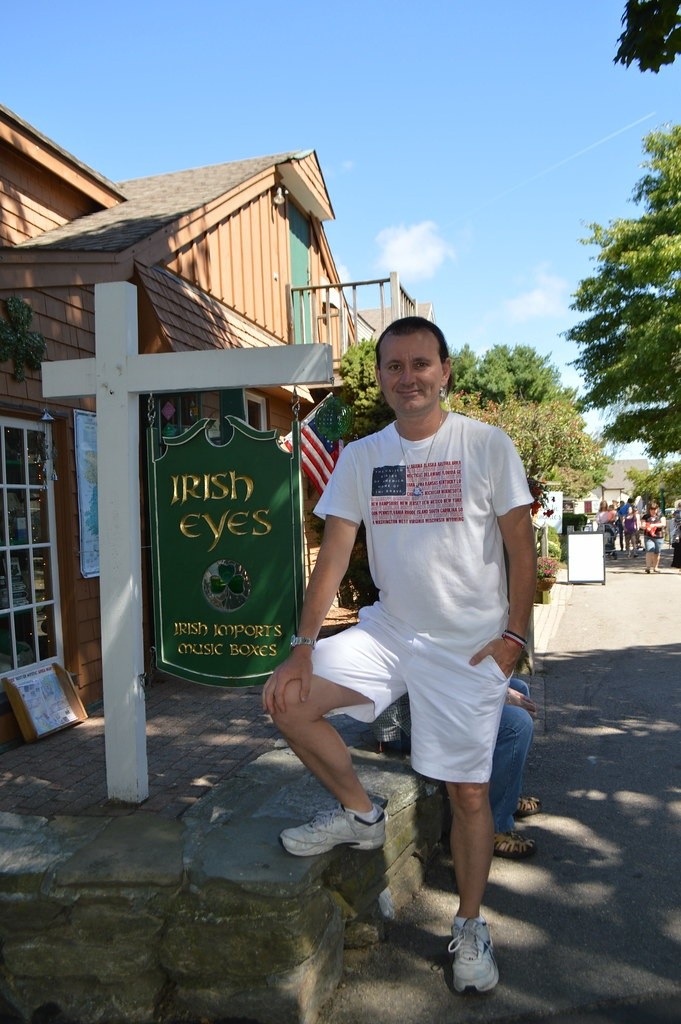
[[493, 831, 537, 858], [514, 796, 543, 814]]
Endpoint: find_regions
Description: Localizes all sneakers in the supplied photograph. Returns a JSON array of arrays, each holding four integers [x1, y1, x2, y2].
[[278, 803, 386, 857], [447, 917, 499, 994]]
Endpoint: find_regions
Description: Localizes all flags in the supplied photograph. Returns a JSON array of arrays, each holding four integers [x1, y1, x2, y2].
[[282, 392, 358, 495]]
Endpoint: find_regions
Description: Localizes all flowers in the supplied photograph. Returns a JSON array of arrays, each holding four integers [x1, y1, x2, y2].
[[537, 556, 560, 579]]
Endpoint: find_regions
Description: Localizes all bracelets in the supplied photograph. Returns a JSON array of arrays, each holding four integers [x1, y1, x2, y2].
[[501, 628, 529, 651]]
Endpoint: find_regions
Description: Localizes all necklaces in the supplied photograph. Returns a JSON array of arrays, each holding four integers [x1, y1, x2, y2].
[[399, 410, 443, 495]]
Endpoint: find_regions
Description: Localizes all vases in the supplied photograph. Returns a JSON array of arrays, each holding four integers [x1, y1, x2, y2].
[[536, 578, 558, 592]]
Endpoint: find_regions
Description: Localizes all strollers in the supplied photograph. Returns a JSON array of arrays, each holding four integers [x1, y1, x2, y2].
[[597, 522, 619, 560]]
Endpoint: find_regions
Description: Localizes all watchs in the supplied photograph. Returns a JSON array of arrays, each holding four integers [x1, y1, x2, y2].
[[290, 634, 317, 650]]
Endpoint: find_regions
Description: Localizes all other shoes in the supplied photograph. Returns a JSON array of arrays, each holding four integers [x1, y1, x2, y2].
[[654, 570, 660, 572], [645, 569, 650, 574]]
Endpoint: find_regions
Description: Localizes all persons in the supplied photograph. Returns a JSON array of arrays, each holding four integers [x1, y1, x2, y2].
[[596, 498, 681, 574], [262, 318, 537, 997], [371, 656, 541, 860]]
